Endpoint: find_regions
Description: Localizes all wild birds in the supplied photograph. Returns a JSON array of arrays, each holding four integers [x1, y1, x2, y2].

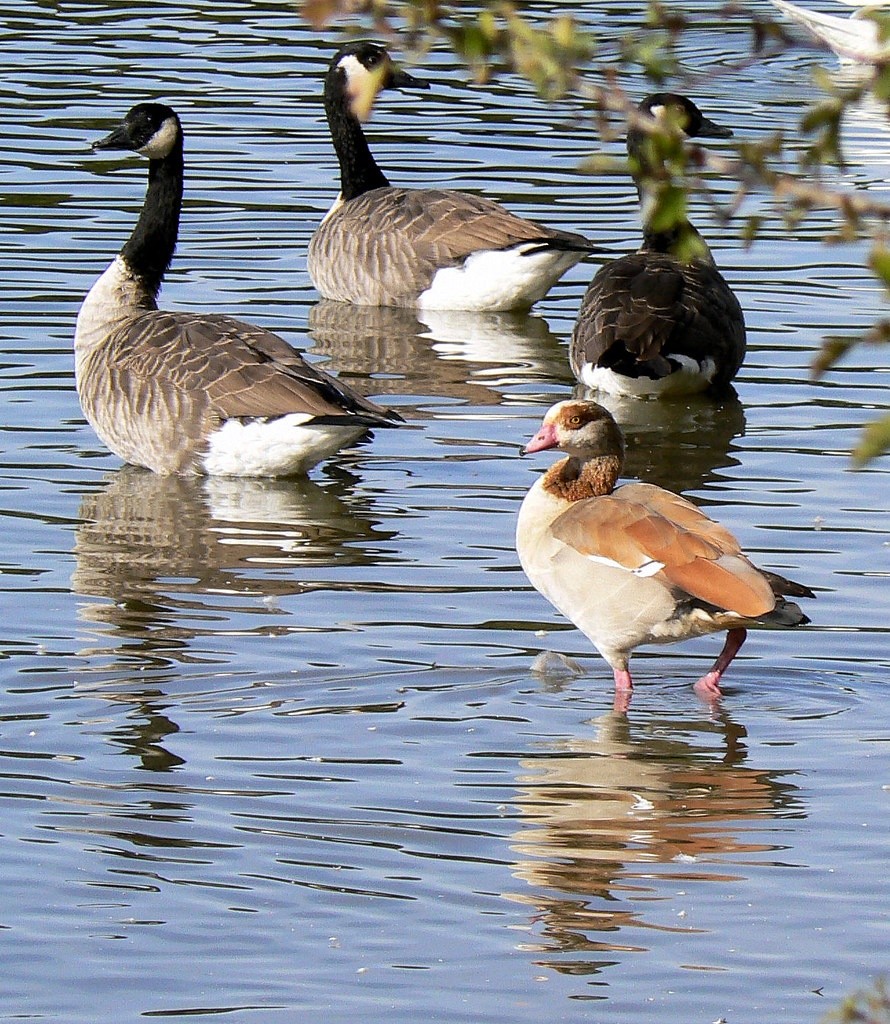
[[774, 0, 890, 64], [515, 400, 815, 698], [569, 92, 747, 396], [73, 102, 406, 478], [305, 40, 610, 313]]
